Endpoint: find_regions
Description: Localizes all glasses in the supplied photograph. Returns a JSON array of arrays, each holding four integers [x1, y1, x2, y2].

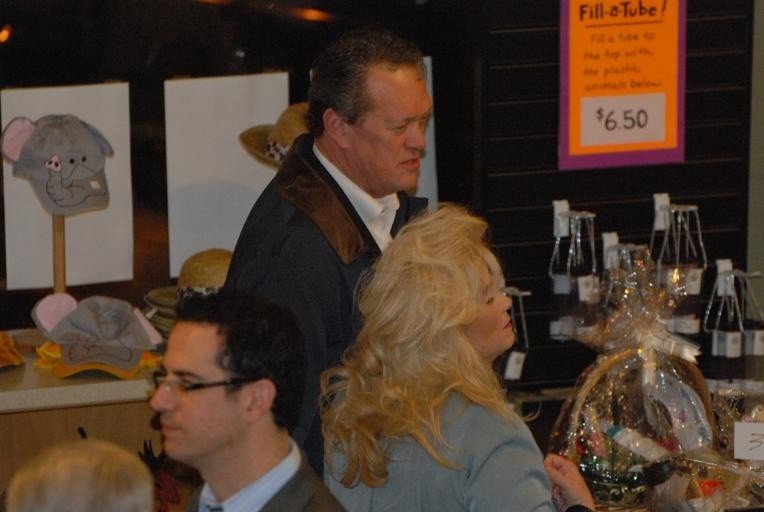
[[145, 371, 264, 396]]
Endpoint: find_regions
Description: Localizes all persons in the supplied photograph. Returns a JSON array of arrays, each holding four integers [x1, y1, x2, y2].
[[146, 286, 349, 512], [219, 25, 432, 480], [315, 200, 596, 512], [3, 439, 158, 512]]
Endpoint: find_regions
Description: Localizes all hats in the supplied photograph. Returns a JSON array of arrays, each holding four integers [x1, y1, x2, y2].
[[239, 101, 313, 172], [29, 288, 162, 381], [1, 109, 121, 220], [141, 246, 237, 340]]
